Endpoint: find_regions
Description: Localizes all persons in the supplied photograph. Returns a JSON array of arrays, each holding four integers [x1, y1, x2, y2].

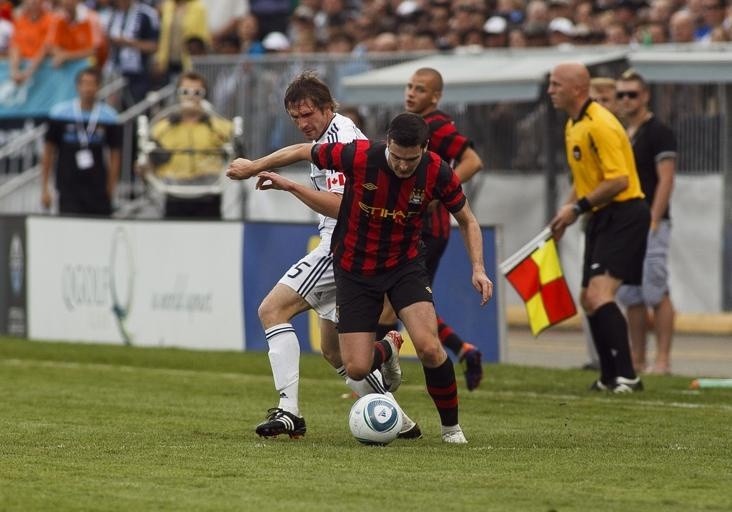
[[374, 66, 485, 395], [141, 72, 233, 221], [615, 65, 678, 377], [226, 108, 495, 448], [2, 1, 260, 86], [544, 60, 650, 395], [573, 72, 629, 372], [251, 75, 423, 441], [235, 1, 731, 170], [40, 68, 124, 217]]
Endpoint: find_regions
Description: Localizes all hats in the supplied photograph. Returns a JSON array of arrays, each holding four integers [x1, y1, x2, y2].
[[548, 17, 575, 38]]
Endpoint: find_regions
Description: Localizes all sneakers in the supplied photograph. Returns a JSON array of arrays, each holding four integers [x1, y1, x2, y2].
[[380, 330, 403, 392], [589, 374, 644, 398], [442, 429, 468, 445], [397, 422, 422, 440], [255, 408, 306, 440], [460, 343, 483, 391]]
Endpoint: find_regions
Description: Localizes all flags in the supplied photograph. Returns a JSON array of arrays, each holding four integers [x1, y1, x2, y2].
[[501, 229, 578, 335]]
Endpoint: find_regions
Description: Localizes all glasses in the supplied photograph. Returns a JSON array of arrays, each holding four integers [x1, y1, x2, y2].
[[616, 91, 637, 99]]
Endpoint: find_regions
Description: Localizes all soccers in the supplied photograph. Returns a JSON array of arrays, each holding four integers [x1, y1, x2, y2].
[[349, 393, 401, 446]]
